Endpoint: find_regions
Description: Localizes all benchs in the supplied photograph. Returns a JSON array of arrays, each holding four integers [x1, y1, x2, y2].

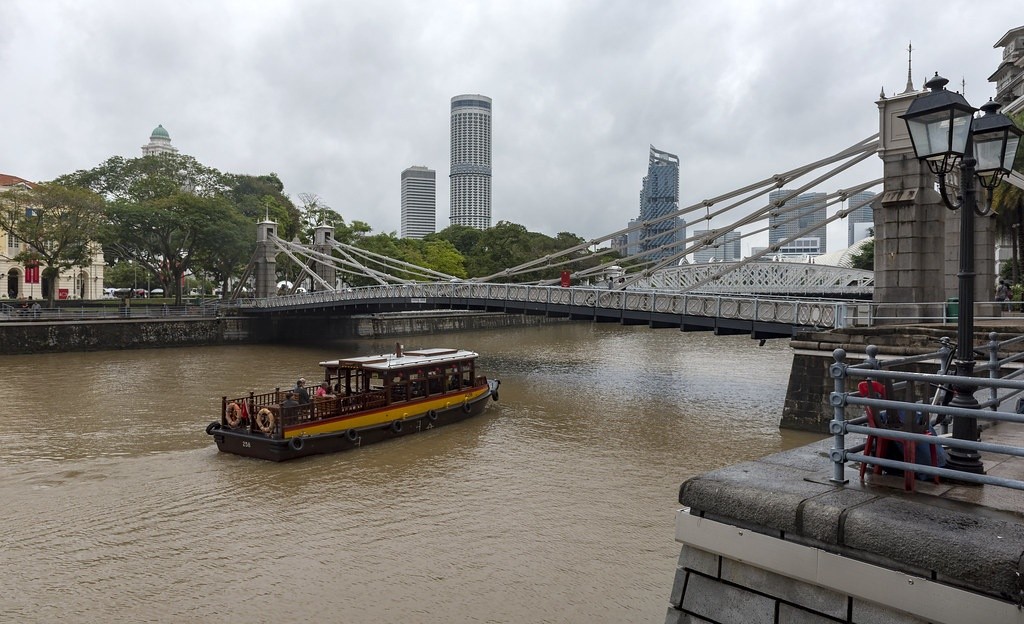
[[279, 391, 300, 404], [390, 386, 406, 400], [314, 396, 335, 412]]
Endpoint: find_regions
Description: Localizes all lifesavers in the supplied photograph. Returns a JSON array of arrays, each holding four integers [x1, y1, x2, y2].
[[492, 390, 499, 401], [206, 422, 220, 434], [428, 409, 438, 421], [257, 408, 274, 431], [392, 420, 403, 433], [463, 402, 471, 414], [289, 436, 304, 453], [346, 428, 357, 441], [226, 403, 241, 426]]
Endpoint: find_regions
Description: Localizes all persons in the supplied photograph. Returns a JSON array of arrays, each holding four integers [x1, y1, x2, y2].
[[316, 381, 342, 413], [995, 280, 1013, 313], [558, 264, 626, 291], [293, 380, 318, 421], [21, 294, 43, 320], [300, 378, 306, 389], [281, 392, 300, 426]]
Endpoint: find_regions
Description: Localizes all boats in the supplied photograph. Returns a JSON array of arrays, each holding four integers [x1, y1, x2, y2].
[[206, 342, 502, 462]]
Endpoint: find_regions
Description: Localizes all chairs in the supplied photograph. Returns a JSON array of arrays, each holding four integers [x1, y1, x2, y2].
[[857, 380, 940, 494]]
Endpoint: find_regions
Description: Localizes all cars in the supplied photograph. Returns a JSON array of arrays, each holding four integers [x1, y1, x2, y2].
[[102, 287, 165, 300]]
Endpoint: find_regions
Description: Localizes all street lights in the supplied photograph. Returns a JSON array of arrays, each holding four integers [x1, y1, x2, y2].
[[895, 70, 1023, 488]]
